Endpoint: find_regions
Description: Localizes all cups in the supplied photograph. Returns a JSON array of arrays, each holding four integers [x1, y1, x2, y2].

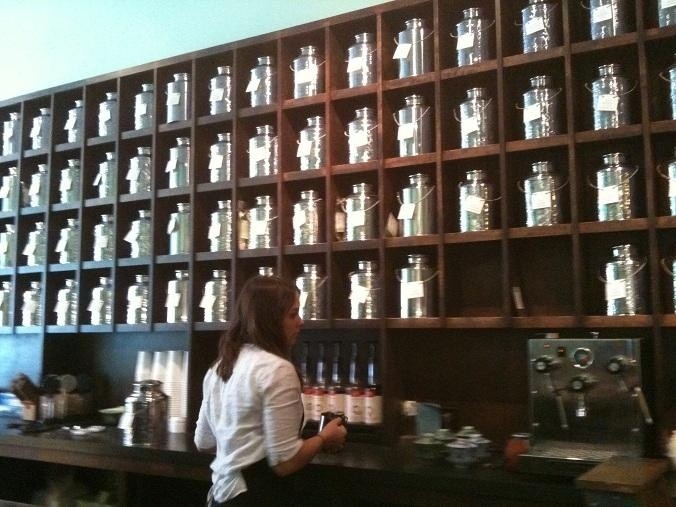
[[315, 411, 349, 451], [412, 425, 496, 469], [132, 348, 188, 434]]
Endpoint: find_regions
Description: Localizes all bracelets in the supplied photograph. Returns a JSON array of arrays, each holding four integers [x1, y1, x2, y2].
[[314, 432, 327, 452]]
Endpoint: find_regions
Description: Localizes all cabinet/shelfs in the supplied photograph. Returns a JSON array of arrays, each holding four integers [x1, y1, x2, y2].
[[1, 0, 676, 334]]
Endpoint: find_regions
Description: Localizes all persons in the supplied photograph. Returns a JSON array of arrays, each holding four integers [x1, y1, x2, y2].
[[191, 276, 347, 507]]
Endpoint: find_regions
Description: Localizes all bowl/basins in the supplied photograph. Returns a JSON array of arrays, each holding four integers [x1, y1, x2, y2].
[[98, 408, 124, 426]]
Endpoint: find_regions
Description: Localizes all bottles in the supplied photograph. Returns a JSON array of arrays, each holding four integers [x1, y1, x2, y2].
[[85, 70, 193, 325], [20, 390, 82, 422], [198, 44, 328, 323], [289, 340, 383, 433], [0, 99, 82, 327], [124, 380, 169, 448], [399, 400, 419, 440], [578, 0, 676, 318], [448, 0, 568, 234], [336, 16, 438, 319], [503, 432, 535, 473]]
